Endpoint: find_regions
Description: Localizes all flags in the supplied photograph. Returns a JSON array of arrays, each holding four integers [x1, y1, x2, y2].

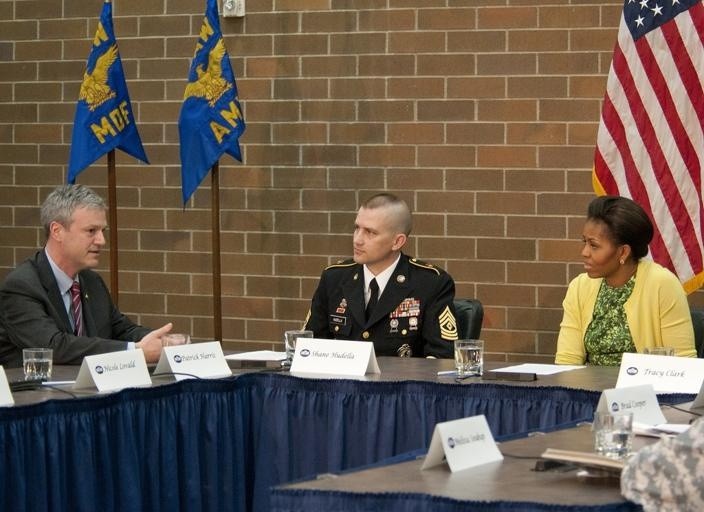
[[67, 1, 149, 185], [179, 0, 245, 212], [592, 0, 704, 299]]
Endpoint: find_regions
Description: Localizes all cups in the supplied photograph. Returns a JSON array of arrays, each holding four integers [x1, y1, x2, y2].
[[452, 340, 483, 378], [593, 413, 633, 456], [644, 346, 675, 357], [21, 346, 53, 381], [162, 333, 190, 347], [282, 329, 314, 363]]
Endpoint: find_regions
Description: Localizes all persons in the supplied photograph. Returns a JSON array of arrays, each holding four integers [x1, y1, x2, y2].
[[301, 194, 462, 359], [1, 183, 175, 370], [554, 196, 699, 371]]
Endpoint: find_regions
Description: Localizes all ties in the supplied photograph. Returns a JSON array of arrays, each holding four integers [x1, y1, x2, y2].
[[71, 282, 82, 337], [365, 278, 378, 323]]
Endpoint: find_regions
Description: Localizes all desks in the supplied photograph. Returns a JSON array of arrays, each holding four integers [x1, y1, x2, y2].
[[0, 350, 697, 512]]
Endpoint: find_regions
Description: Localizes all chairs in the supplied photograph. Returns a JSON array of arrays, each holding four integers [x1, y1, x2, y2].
[[454, 297, 483, 340], [690, 307, 704, 358]]
